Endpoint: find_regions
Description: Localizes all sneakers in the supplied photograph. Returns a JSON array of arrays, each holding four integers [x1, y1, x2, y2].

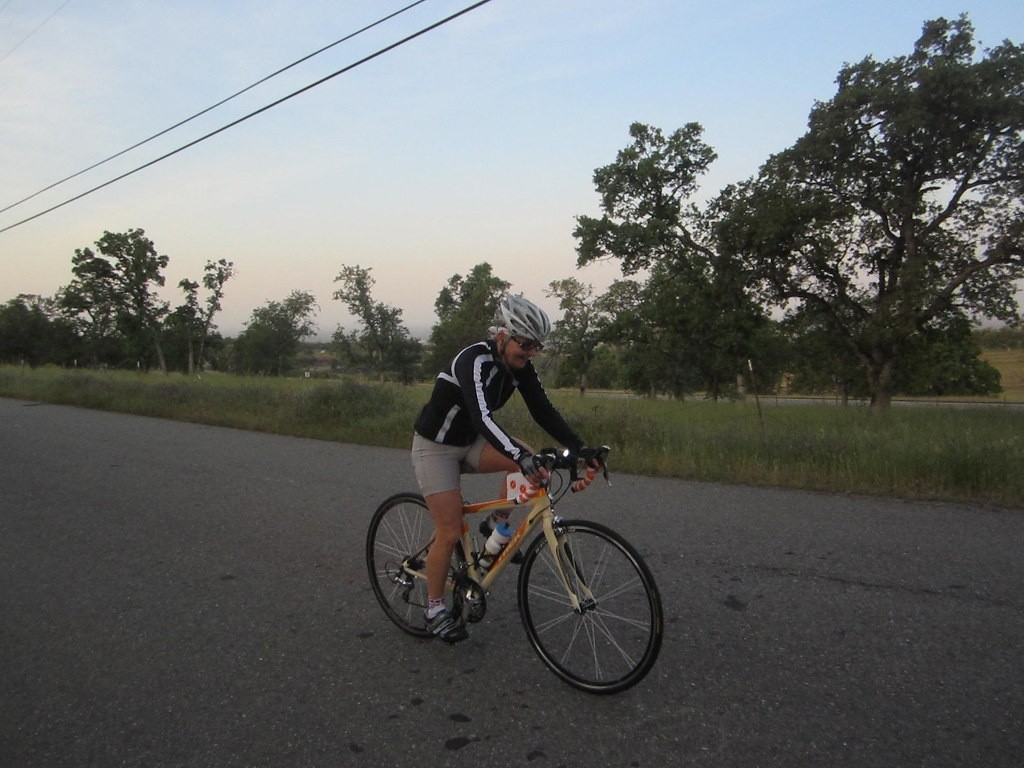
[[479, 516, 523, 564], [422, 607, 468, 643]]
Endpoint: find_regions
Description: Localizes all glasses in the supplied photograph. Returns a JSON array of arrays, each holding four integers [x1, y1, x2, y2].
[[512, 337, 545, 352]]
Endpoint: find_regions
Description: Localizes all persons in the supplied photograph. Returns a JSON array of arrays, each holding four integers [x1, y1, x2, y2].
[[411, 294, 604, 643]]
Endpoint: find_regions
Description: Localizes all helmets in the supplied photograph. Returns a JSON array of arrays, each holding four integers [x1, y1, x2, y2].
[[487, 294, 551, 344]]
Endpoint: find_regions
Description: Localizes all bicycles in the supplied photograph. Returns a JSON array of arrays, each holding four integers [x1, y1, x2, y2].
[[366, 446, 664, 696]]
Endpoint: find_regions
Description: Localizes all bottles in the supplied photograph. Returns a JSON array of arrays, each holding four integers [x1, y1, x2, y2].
[[477, 523, 513, 568]]
